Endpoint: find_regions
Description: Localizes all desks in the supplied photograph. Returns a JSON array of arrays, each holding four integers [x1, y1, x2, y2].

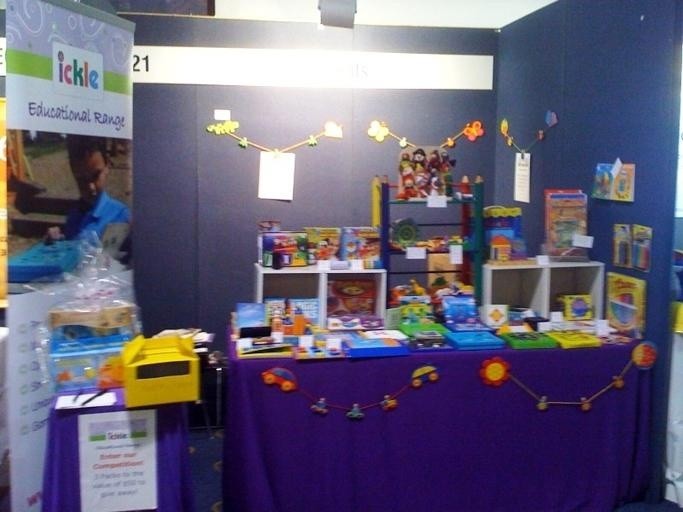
[[226, 312, 643, 512]]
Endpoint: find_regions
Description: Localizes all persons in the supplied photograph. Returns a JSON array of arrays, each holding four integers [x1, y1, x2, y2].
[[396, 148, 472, 200], [43, 135, 131, 262]]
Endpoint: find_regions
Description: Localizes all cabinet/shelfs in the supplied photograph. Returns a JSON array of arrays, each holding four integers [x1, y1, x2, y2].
[[254, 263, 389, 328], [483, 258, 606, 323]]
